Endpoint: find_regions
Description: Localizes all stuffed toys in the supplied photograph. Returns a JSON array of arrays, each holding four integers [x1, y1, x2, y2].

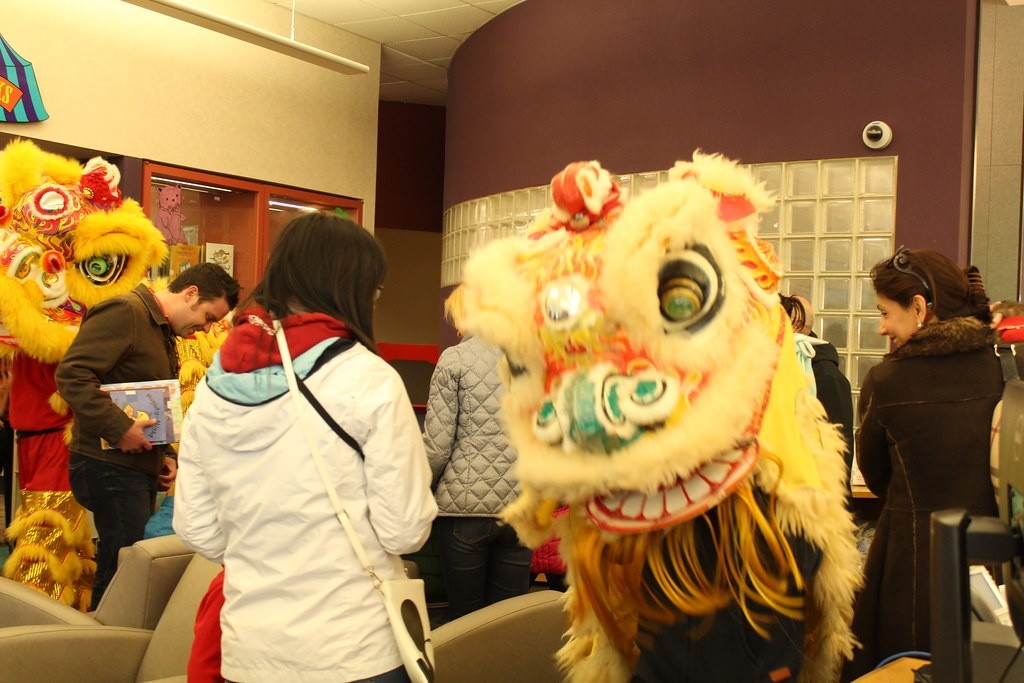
[[1, 137, 236, 499], [445, 147, 866, 682]]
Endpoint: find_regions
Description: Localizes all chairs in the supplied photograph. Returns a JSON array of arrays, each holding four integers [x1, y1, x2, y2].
[[0, 530, 569, 682]]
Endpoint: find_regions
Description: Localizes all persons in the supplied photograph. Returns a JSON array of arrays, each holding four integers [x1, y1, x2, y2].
[[629, 485, 821, 682], [56, 261, 244, 610], [418, 332, 531, 622], [2, 349, 99, 617], [779, 294, 855, 515], [173, 215, 440, 681], [845, 250, 1007, 677]]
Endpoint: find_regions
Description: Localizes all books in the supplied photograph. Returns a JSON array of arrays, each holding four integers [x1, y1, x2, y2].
[[96, 378, 186, 450]]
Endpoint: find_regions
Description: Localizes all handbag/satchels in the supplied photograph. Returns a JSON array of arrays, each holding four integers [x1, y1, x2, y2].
[[379, 579, 435, 683]]
